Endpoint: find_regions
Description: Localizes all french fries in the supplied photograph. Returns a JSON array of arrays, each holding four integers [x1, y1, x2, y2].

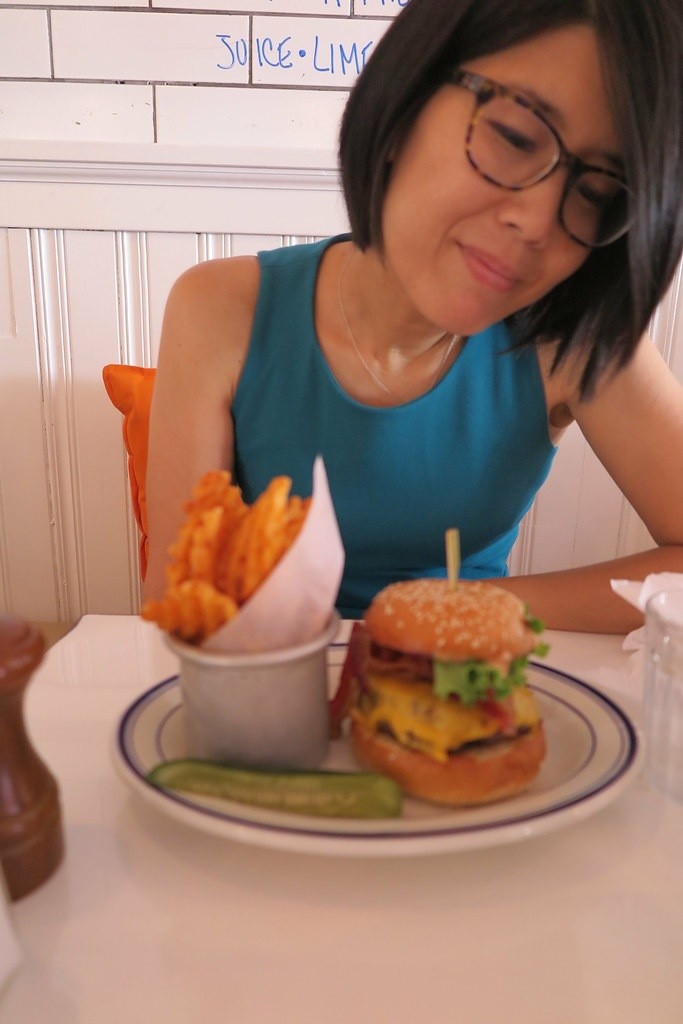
[[138, 468, 312, 647]]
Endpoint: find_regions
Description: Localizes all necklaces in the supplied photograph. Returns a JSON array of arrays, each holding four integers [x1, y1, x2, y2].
[[338, 247, 457, 405]]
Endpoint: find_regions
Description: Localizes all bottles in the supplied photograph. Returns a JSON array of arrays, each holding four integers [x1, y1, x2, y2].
[[0, 618, 62, 904]]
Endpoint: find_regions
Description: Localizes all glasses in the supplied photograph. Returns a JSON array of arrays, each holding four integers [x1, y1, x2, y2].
[[440, 63, 642, 249]]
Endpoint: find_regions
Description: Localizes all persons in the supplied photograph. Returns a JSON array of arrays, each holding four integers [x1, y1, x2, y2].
[[142, 0, 682, 635]]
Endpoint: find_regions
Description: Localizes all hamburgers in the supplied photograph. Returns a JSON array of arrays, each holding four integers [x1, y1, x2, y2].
[[345, 578, 551, 805]]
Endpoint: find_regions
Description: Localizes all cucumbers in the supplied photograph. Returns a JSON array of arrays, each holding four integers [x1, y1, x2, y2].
[[149, 757, 403, 821]]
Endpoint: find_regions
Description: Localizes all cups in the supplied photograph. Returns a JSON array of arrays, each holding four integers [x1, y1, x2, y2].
[[640, 589, 683, 804], [162, 605, 340, 767]]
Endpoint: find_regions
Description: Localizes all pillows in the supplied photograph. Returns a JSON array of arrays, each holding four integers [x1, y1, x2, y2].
[[103, 364, 157, 582]]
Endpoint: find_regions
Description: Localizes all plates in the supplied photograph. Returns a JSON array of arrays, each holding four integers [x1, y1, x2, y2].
[[112, 641, 643, 855]]
